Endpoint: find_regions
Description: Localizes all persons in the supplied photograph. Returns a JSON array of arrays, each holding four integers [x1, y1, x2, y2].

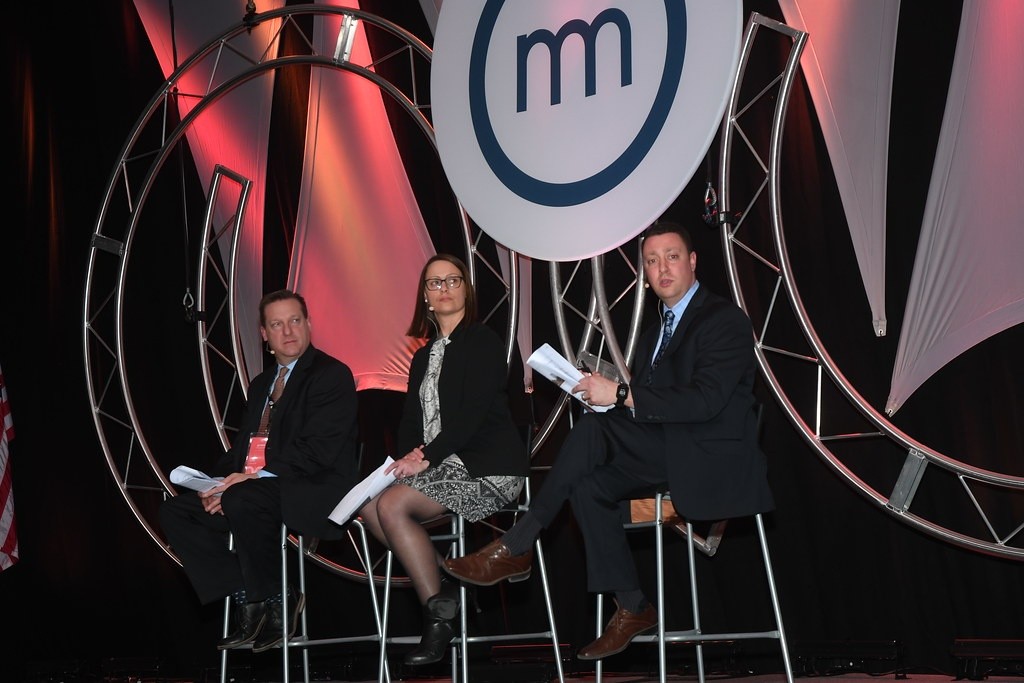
[[439, 223, 778, 660], [156, 288, 357, 654], [354, 253, 532, 667]]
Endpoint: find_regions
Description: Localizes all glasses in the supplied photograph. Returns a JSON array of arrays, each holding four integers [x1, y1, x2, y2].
[[418, 274, 465, 292]]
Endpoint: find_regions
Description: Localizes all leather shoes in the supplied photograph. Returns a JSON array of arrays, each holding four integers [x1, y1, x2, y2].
[[216, 581, 302, 651], [440, 540, 532, 587], [576, 602, 660, 658]]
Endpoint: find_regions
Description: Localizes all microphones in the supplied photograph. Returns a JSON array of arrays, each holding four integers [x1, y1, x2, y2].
[[266, 342, 275, 354], [429, 307, 434, 311], [645, 284, 650, 288]]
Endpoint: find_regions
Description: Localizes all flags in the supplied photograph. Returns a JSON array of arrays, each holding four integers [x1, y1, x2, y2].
[[0, 373, 26, 576]]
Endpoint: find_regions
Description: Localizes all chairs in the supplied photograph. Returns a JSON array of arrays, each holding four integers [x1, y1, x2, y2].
[[374, 392, 565, 683], [219, 438, 392, 683]]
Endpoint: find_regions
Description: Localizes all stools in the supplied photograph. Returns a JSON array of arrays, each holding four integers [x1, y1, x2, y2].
[[594, 491, 795, 683]]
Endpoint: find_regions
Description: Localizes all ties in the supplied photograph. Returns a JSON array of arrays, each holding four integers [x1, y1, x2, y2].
[[654, 312, 676, 368], [253, 368, 289, 435]]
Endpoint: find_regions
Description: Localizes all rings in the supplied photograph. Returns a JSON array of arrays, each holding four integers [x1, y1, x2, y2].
[[402, 474, 407, 478], [582, 393, 586, 401]]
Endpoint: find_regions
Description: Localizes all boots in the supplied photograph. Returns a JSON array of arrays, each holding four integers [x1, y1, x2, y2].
[[404, 572, 480, 669]]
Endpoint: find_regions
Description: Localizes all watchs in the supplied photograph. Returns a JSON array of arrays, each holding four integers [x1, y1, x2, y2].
[[615, 382, 629, 410]]
